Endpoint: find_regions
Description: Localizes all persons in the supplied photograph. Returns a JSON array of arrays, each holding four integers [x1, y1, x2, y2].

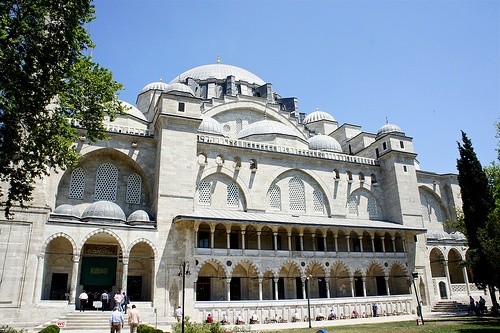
[[78, 290, 88, 312], [220, 313, 228, 325], [372, 304, 378, 317], [203, 313, 214, 325], [468, 295, 500, 317], [114, 290, 124, 310], [121, 292, 129, 314], [109, 306, 124, 333], [235, 314, 243, 325], [264, 308, 407, 325], [249, 313, 258, 324], [87, 291, 115, 311], [416, 301, 423, 325], [175, 306, 182, 322], [127, 305, 140, 333]]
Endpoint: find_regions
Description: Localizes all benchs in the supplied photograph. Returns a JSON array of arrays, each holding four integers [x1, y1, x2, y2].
[[280, 320, 287, 323], [222, 321, 230, 325], [237, 321, 245, 325], [250, 321, 260, 324], [292, 319, 301, 322], [316, 317, 324, 321], [264, 319, 273, 324]]
